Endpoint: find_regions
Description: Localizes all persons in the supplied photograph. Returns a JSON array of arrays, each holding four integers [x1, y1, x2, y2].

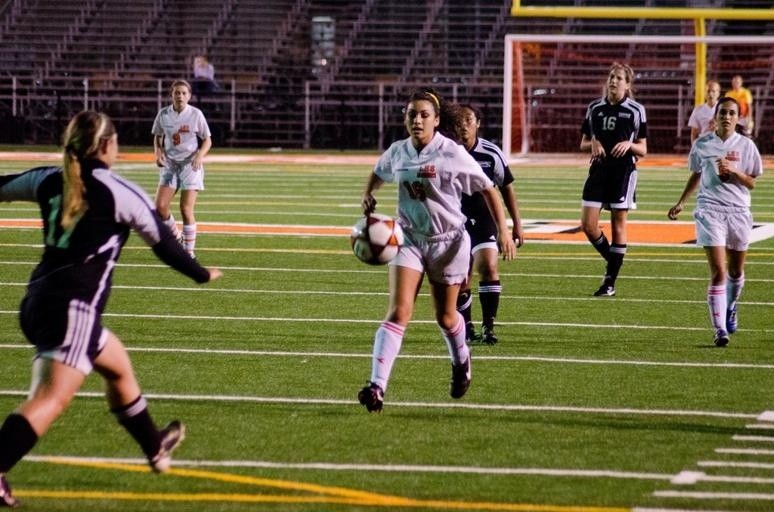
[[579, 61, 647, 296], [455, 101, 524, 347], [0, 110, 222, 507], [357, 90, 516, 412], [151, 80, 212, 260], [668, 100, 764, 346], [686, 80, 724, 149], [191, 57, 214, 82], [724, 74, 756, 141]]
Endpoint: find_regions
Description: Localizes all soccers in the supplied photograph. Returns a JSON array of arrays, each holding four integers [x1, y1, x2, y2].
[[351, 213, 404, 265]]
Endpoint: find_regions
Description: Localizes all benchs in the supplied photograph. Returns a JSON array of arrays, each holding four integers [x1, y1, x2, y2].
[[0, 0, 774, 153]]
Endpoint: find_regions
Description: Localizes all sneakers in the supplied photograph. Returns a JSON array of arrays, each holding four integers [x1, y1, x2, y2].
[[358, 382, 384, 413], [450, 351, 472, 398], [0, 472, 20, 508], [713, 328, 729, 346], [466, 325, 481, 342], [481, 326, 498, 345], [593, 283, 616, 297], [147, 419, 185, 474], [726, 303, 737, 333]]
